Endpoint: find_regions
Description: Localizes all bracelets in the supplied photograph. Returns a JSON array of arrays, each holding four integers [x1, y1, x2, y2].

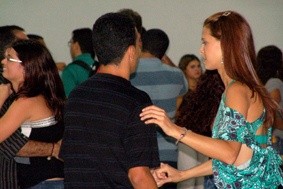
[[177, 126, 189, 145], [48, 143, 55, 161]]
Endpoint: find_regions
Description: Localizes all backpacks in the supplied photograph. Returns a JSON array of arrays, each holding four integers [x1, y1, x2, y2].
[[67, 59, 99, 78]]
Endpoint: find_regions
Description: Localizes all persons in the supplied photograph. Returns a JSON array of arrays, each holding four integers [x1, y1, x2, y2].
[[0, 0, 283, 189]]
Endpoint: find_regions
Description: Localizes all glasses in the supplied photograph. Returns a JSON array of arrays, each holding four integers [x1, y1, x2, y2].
[[3, 54, 22, 63], [68, 40, 73, 46]]
